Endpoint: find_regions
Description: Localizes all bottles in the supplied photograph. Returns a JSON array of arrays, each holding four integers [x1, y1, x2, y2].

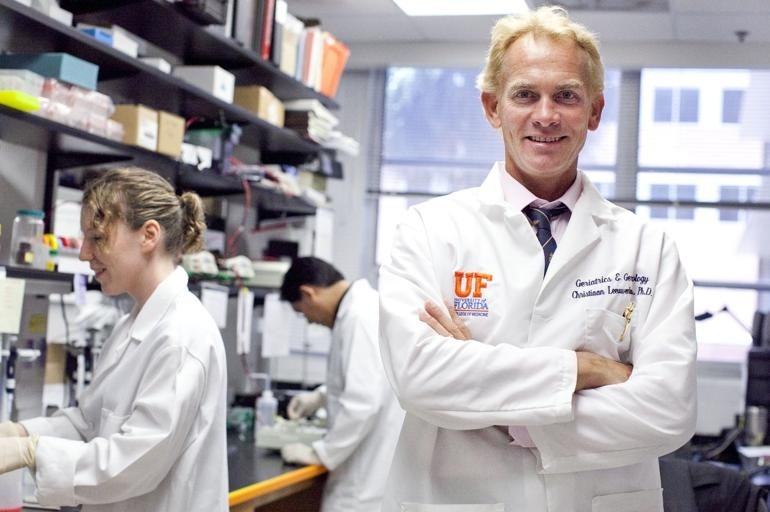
[[255, 390, 277, 443], [9, 209, 46, 270]]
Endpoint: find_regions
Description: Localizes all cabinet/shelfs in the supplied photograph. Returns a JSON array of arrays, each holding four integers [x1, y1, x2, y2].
[[1, 0, 341, 305]]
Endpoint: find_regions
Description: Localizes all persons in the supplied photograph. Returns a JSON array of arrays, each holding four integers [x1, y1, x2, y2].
[[0, 166, 230, 511], [280, 255, 405, 512], [378, 4, 695, 510]]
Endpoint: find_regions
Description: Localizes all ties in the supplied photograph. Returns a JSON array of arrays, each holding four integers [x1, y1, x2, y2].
[[523, 203, 568, 276]]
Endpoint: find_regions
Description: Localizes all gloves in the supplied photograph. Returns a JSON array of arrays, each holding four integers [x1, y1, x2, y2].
[[281, 443, 321, 465], [0, 420, 39, 475], [287, 390, 323, 419]]
